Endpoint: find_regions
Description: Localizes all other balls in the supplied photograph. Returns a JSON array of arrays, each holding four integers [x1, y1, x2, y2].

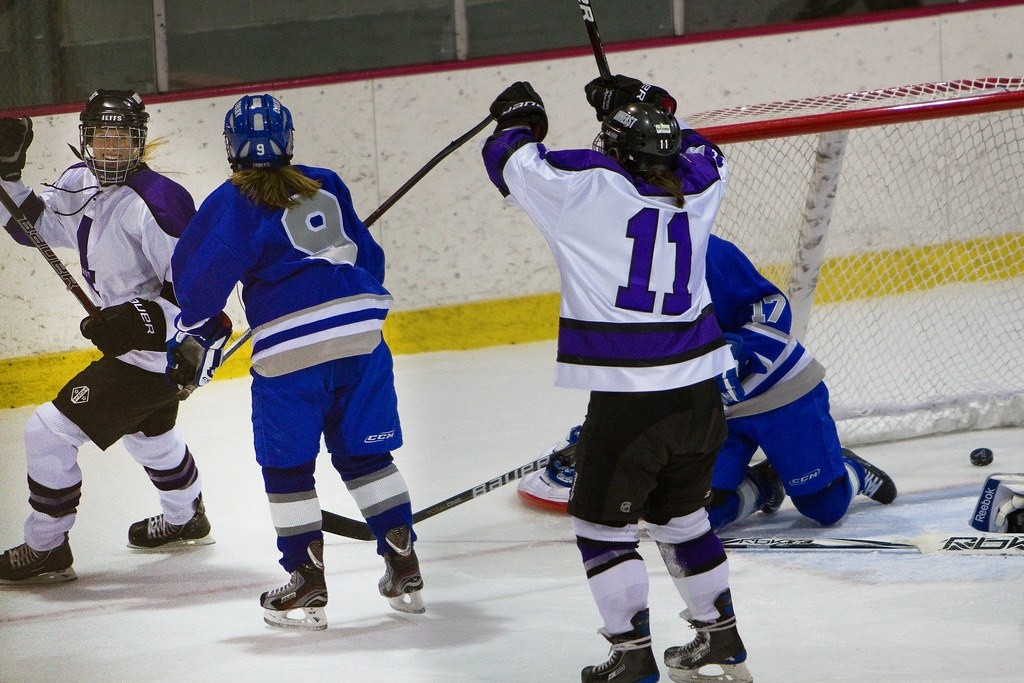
[[970, 448, 993, 466]]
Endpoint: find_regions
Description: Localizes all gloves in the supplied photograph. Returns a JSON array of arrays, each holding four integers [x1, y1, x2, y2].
[[1, 115, 34, 181], [164, 307, 231, 387], [584, 73, 677, 124], [489, 79, 549, 143], [80, 297, 167, 358]]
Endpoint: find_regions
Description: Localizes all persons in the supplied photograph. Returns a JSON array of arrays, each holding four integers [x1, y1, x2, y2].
[[481, 76, 756, 683], [550, 236, 902, 528], [174, 95, 425, 629], [0, 91, 234, 593]]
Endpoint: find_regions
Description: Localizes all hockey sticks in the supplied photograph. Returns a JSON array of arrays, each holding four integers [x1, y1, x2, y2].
[[0, 185, 99, 319], [176, 114, 496, 400], [577, 0, 612, 84], [321, 443, 576, 541], [721, 536, 1024, 556]]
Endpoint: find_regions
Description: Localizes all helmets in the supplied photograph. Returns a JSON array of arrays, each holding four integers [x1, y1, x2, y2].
[[592, 101, 680, 179], [222, 92, 295, 170], [77, 88, 150, 185]]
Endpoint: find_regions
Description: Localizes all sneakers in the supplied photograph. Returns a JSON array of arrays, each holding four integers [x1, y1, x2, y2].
[[260, 538, 326, 630], [126, 491, 216, 550], [378, 524, 427, 615], [0, 531, 80, 585], [664, 588, 754, 683], [581, 607, 659, 683], [748, 458, 786, 514], [840, 444, 897, 502]]
